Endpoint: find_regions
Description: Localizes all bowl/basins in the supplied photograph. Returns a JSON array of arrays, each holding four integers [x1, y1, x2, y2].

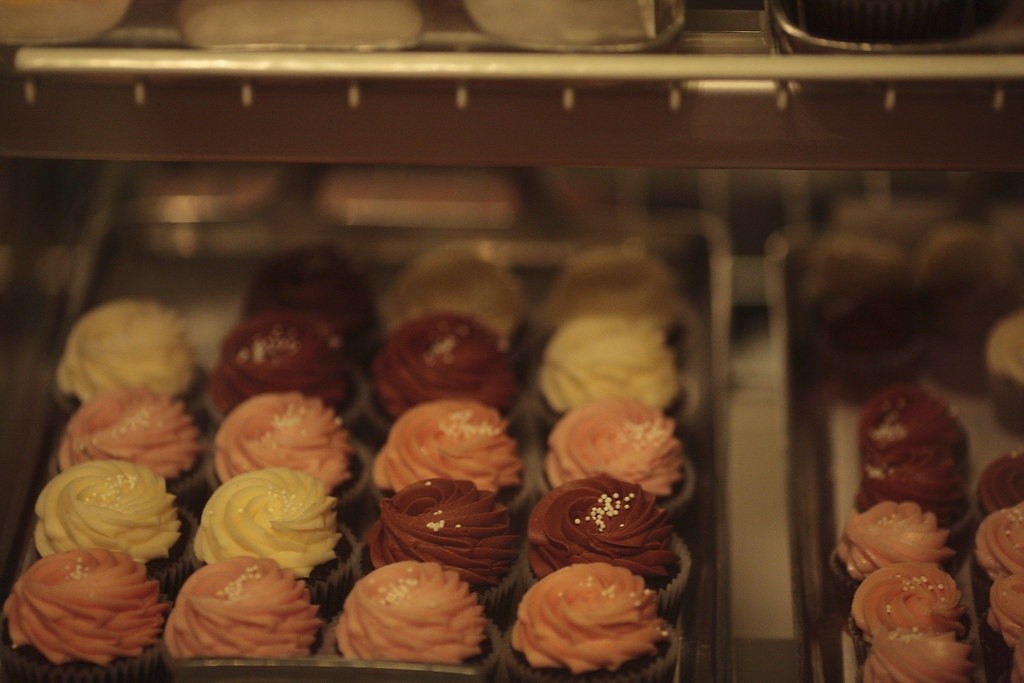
[[761, 0, 1017, 51]]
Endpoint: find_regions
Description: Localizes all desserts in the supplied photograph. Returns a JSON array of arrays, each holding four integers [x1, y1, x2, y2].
[[4, 252, 1020, 682]]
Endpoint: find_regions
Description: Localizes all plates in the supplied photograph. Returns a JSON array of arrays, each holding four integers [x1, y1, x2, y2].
[[2, 2, 132, 45], [464, 2, 686, 52], [175, 1, 423, 50]]
